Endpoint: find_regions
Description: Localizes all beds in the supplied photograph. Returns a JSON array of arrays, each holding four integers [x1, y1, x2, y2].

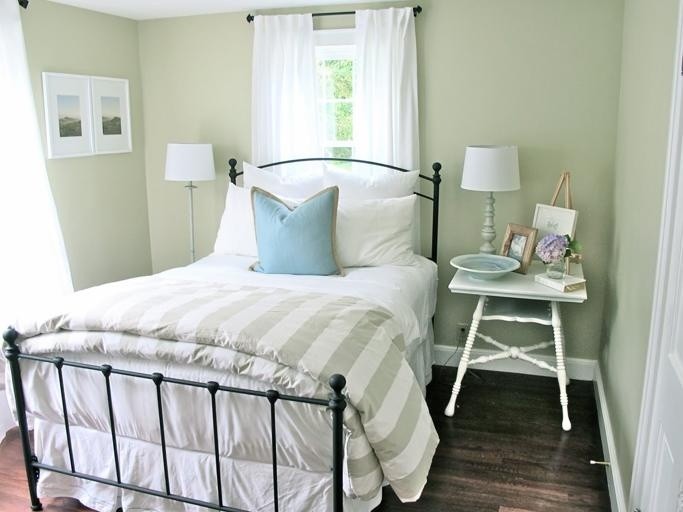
[[2, 158, 441, 511]]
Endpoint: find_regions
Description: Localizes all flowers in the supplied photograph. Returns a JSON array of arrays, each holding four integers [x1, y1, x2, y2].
[[537, 233, 576, 266]]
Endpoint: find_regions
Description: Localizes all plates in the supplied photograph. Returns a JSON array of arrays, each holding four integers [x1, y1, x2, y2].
[[449, 253, 521, 278]]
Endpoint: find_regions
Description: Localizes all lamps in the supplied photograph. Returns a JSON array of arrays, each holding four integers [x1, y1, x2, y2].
[[164, 145, 218, 262], [460, 144, 522, 257]]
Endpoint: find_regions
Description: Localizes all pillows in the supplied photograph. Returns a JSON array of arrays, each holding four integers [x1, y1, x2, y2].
[[217, 160, 422, 277]]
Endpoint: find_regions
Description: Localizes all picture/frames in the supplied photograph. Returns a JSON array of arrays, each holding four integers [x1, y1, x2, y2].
[[501, 224, 538, 275], [41, 69, 133, 160]]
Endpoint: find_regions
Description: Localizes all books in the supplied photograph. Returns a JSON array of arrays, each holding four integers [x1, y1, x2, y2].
[[535, 270, 587, 294]]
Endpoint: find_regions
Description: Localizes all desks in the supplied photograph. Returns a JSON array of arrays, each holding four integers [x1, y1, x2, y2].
[[444, 263, 589, 431]]
